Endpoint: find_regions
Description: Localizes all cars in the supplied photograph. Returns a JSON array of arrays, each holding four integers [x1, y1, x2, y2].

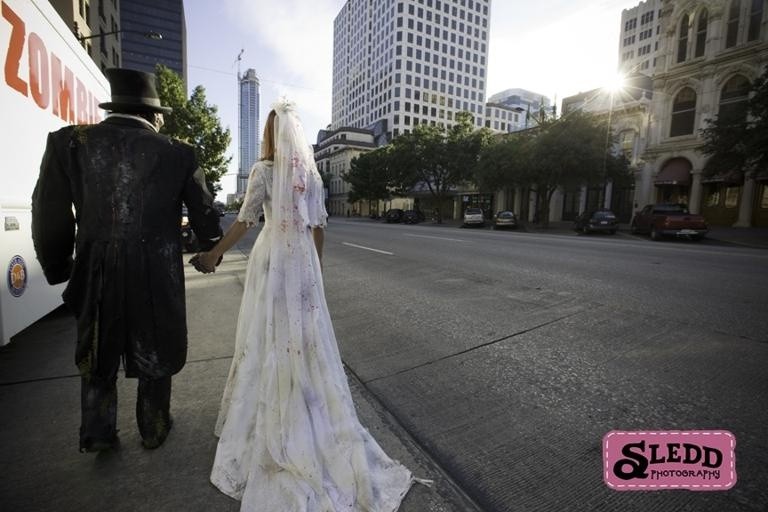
[[404, 210, 426, 225], [572, 209, 620, 236], [631, 203, 712, 241], [494, 209, 516, 227], [463, 207, 485, 227], [386, 209, 404, 224]]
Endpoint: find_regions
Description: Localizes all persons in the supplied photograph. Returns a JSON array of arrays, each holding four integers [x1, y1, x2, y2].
[[30, 66, 223, 451], [196, 94, 433, 512]]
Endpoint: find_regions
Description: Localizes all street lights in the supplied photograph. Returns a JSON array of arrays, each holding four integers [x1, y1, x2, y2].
[[595, 73, 628, 209]]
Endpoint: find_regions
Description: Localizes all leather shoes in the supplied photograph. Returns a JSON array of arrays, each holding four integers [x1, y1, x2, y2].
[[79, 428, 120, 452]]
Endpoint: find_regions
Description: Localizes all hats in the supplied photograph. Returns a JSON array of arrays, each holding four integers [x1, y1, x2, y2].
[[99, 66, 174, 115]]
